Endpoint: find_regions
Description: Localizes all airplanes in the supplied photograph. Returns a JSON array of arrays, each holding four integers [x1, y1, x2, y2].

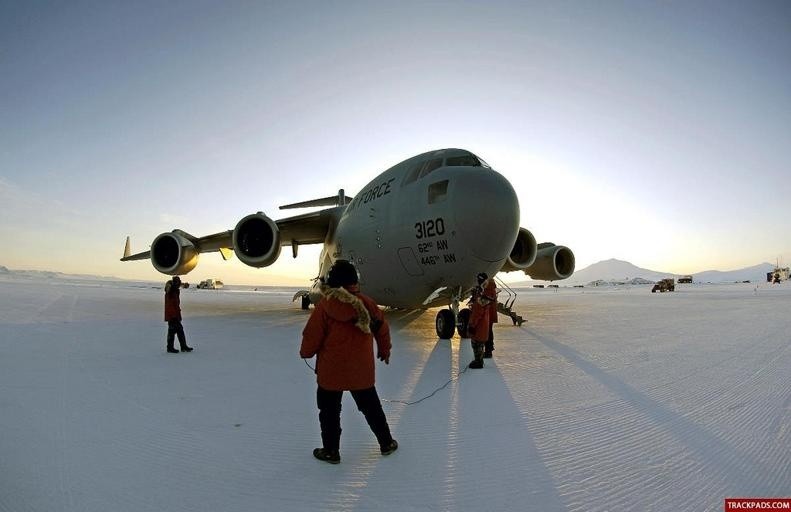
[[118, 148, 575, 340]]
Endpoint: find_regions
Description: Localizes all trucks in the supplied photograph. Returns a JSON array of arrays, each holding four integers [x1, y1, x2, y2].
[[196, 279, 223, 289], [651, 278, 674, 292]]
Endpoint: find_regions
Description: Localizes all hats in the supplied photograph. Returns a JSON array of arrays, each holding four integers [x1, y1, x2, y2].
[[327, 260, 358, 288], [476, 272, 488, 281], [172, 276, 181, 285], [470, 285, 484, 296]]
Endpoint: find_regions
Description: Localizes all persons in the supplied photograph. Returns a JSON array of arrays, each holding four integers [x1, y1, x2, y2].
[[466, 285, 491, 368], [298, 260, 401, 463], [162, 276, 194, 353], [473, 273, 500, 359]]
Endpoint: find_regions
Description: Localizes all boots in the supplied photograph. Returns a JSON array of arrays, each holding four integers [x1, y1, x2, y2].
[[178, 336, 193, 352], [166, 335, 179, 353]]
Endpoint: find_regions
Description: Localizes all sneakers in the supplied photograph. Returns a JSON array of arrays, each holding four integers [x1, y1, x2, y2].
[[380, 440, 398, 455], [313, 448, 340, 464], [469, 351, 492, 369]]
